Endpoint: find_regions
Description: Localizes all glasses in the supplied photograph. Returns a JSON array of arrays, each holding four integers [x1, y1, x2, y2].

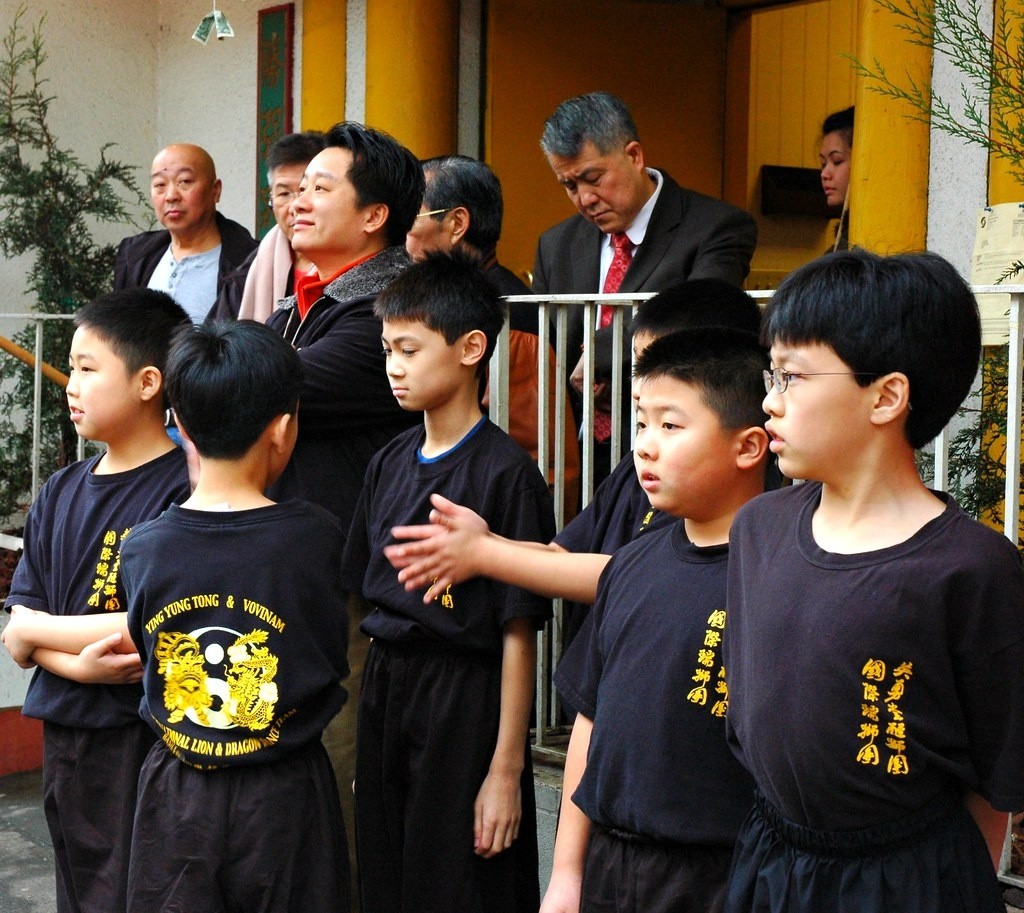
[[763, 367, 913, 411], [268, 189, 299, 207]]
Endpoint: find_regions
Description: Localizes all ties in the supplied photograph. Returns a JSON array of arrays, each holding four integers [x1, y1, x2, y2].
[[594, 232, 630, 443]]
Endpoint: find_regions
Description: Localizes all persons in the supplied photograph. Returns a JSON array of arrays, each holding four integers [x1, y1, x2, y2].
[[112, 119, 580, 542], [818, 104, 857, 255], [532, 92, 761, 515], [384, 278, 762, 607], [0, 290, 197, 913], [534, 329, 771, 913], [118, 320, 352, 913], [342, 250, 555, 913], [723, 247, 1023, 913]]
